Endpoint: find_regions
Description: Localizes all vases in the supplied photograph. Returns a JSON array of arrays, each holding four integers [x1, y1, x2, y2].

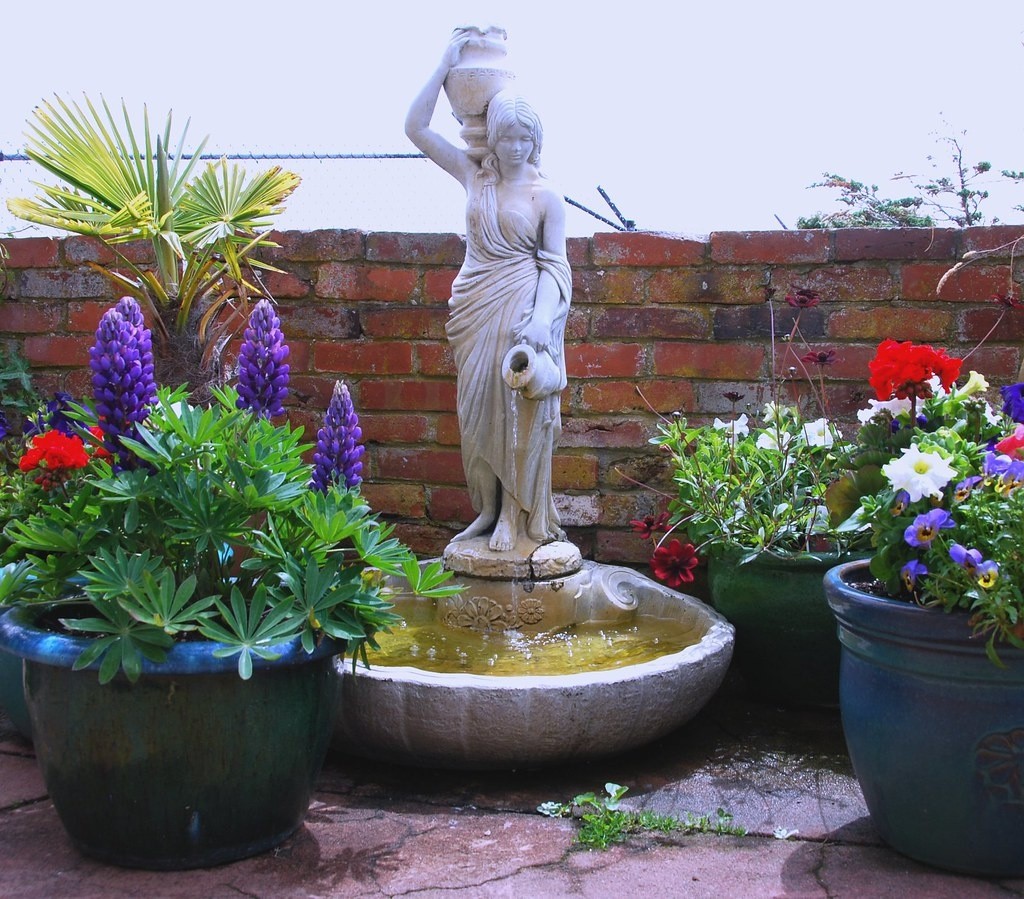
[[820, 559, 1024, 882], [704, 539, 872, 728], [0, 552, 350, 872]]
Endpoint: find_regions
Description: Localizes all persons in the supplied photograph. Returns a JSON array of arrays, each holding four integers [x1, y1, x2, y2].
[[405, 28, 572, 553]]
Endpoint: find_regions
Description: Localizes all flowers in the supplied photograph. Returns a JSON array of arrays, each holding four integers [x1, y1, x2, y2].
[[0, 294, 468, 682], [612, 279, 872, 589], [823, 338, 1023, 670]]
